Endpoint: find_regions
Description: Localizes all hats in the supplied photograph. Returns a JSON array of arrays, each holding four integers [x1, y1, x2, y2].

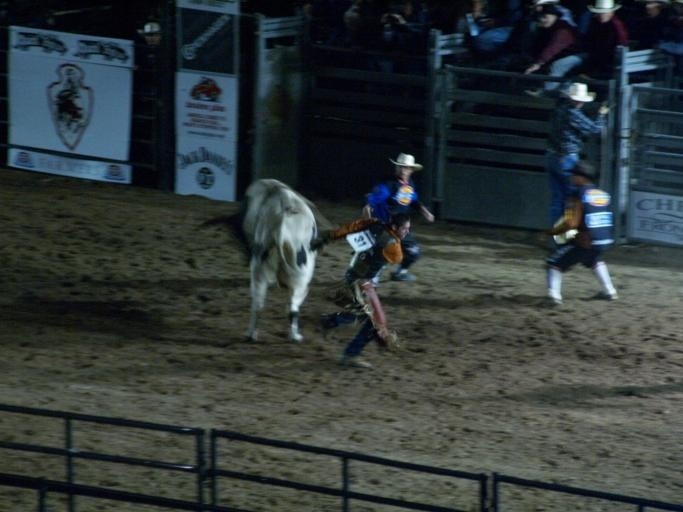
[[588, 0, 622, 14], [563, 161, 596, 176], [530, 4, 563, 22], [389, 153, 423, 171], [137, 22, 160, 34], [558, 82, 597, 102]]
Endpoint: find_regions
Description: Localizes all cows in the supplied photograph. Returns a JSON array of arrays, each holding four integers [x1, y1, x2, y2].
[[196, 174, 323, 346]]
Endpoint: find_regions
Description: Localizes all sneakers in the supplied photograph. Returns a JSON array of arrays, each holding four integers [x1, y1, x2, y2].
[[535, 296, 562, 309], [592, 291, 619, 300], [390, 272, 417, 281], [320, 313, 335, 342], [344, 355, 372, 368]]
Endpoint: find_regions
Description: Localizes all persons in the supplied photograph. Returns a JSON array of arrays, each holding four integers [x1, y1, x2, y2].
[[538, 83, 611, 253], [308, 212, 411, 369], [132, 22, 173, 92], [300, 0, 683, 96], [530, 159, 619, 309], [360, 153, 435, 288]]
